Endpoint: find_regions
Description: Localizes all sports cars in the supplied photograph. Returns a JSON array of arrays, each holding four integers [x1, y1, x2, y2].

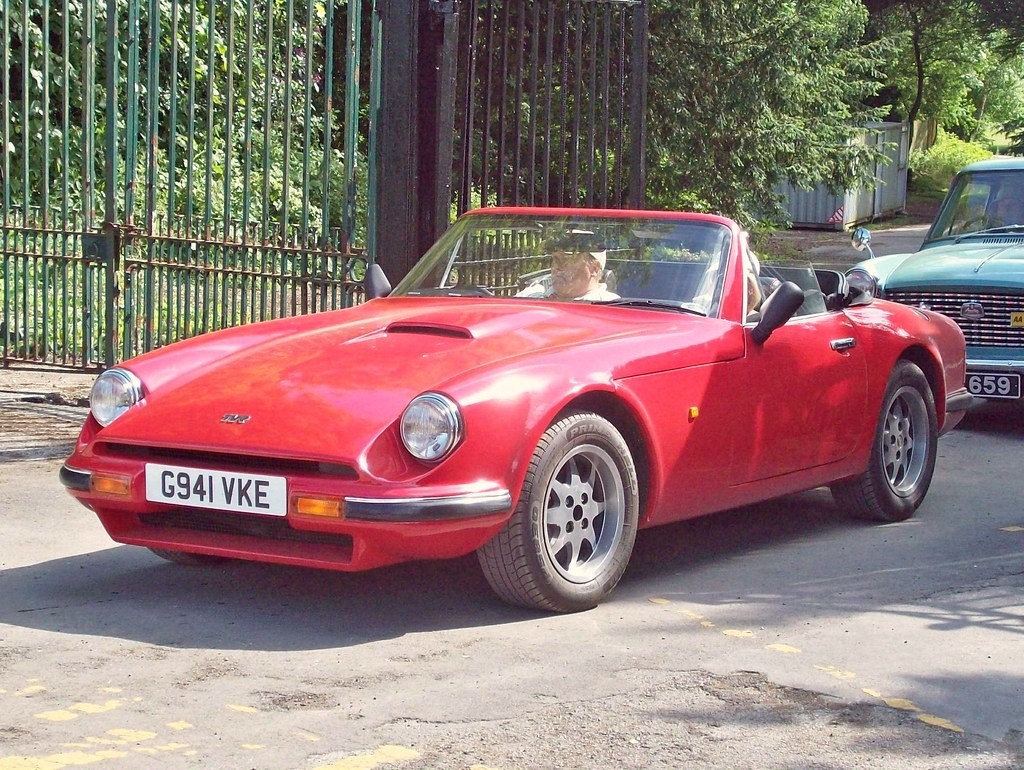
[[58, 209, 974, 615]]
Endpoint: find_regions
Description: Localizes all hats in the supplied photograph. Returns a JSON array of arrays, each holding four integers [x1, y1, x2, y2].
[[561, 229, 606, 270]]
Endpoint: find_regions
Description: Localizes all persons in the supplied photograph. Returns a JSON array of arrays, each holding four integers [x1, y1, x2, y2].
[[513, 230, 621, 301], [956, 184, 1024, 233], [693, 250, 766, 325]]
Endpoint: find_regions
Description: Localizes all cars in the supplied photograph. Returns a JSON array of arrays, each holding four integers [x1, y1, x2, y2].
[[842, 157, 1024, 404]]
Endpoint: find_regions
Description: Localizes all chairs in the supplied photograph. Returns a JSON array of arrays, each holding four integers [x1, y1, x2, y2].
[[598, 269, 617, 293], [753, 277, 797, 320]]
